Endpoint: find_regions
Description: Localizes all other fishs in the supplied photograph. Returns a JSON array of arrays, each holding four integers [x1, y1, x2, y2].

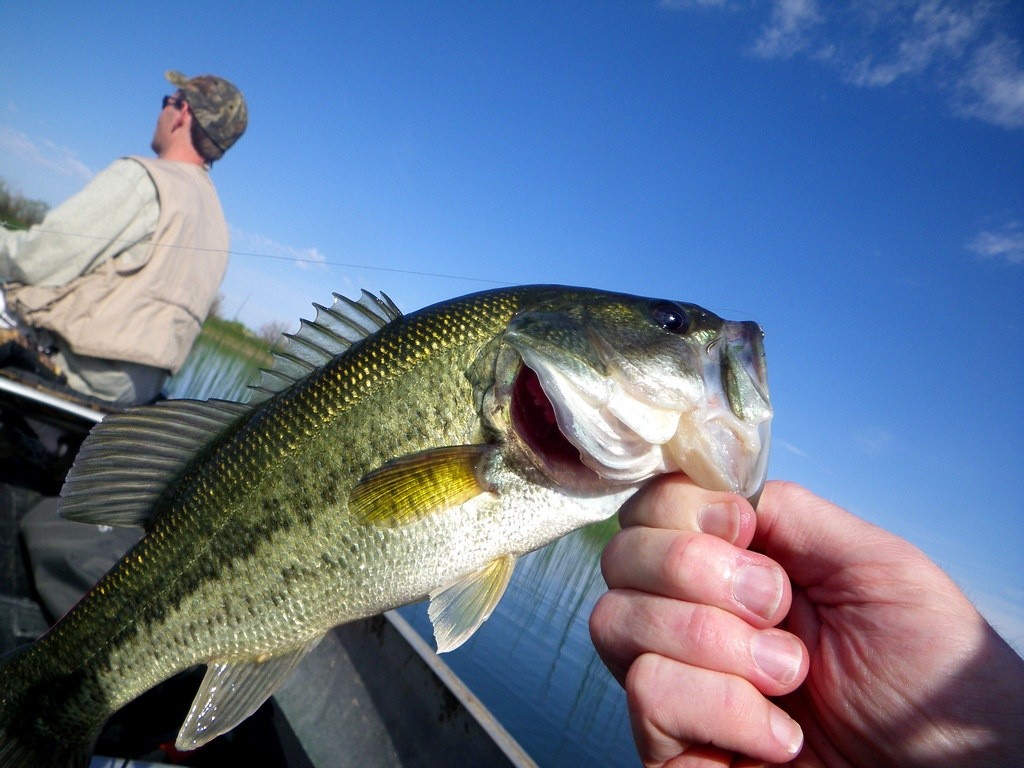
[[1, 285, 774, 768]]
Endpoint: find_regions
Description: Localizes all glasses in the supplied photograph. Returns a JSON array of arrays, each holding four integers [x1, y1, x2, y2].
[[163, 95, 191, 112]]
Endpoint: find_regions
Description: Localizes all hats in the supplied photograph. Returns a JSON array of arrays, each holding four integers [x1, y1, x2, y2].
[[166, 71, 248, 151]]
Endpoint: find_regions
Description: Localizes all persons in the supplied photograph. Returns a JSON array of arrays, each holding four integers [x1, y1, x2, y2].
[[586, 472, 1024, 768], [0, 73, 248, 409]]
